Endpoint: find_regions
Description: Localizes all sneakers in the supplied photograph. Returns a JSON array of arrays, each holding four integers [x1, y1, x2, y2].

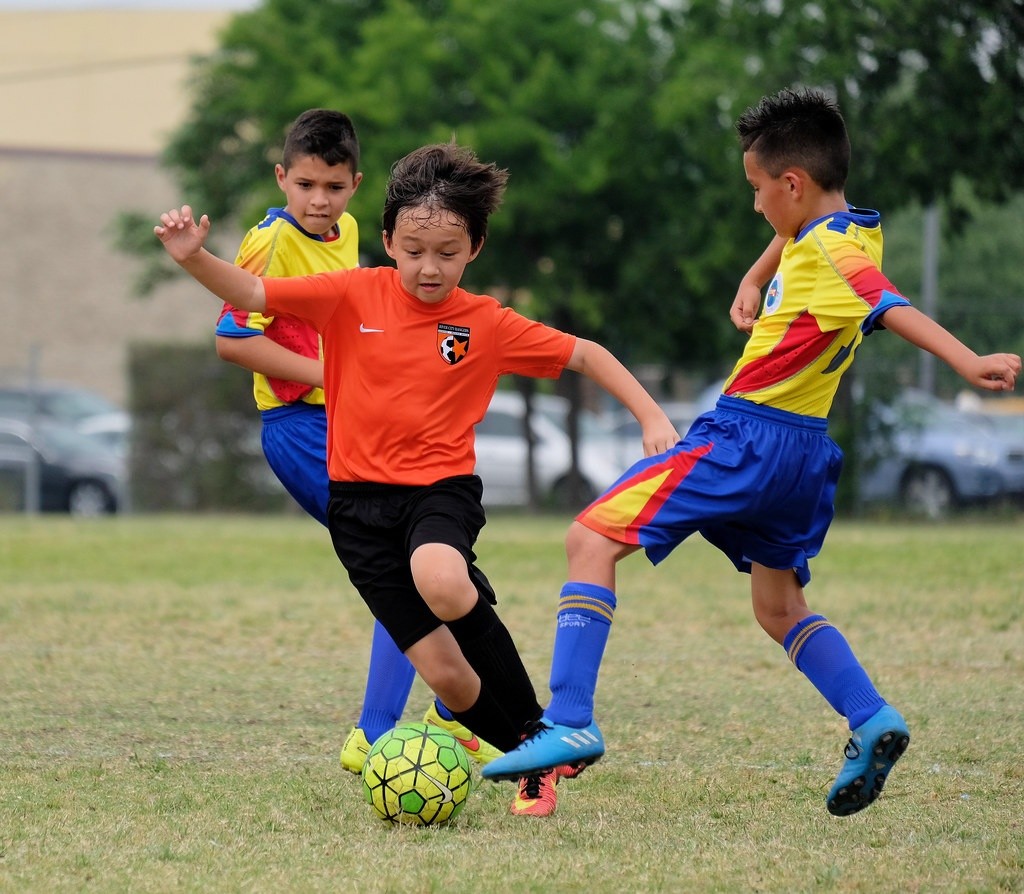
[[828, 697, 911, 817], [518, 708, 586, 779], [481, 720, 604, 783], [339, 726, 373, 775], [423, 701, 505, 764]]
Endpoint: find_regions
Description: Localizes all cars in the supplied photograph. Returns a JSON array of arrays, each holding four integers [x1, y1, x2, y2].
[[1, 381, 133, 515], [470, 391, 626, 509], [595, 375, 1024, 523]]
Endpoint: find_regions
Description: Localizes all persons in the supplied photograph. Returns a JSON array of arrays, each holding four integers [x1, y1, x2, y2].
[[478, 83, 1023, 815], [148, 142, 683, 817], [216, 109, 507, 776]]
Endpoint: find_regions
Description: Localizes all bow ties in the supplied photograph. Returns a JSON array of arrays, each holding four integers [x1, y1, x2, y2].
[[509, 766, 561, 818]]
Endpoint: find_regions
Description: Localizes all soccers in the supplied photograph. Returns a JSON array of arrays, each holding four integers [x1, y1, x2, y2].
[[363, 721, 471, 829]]
